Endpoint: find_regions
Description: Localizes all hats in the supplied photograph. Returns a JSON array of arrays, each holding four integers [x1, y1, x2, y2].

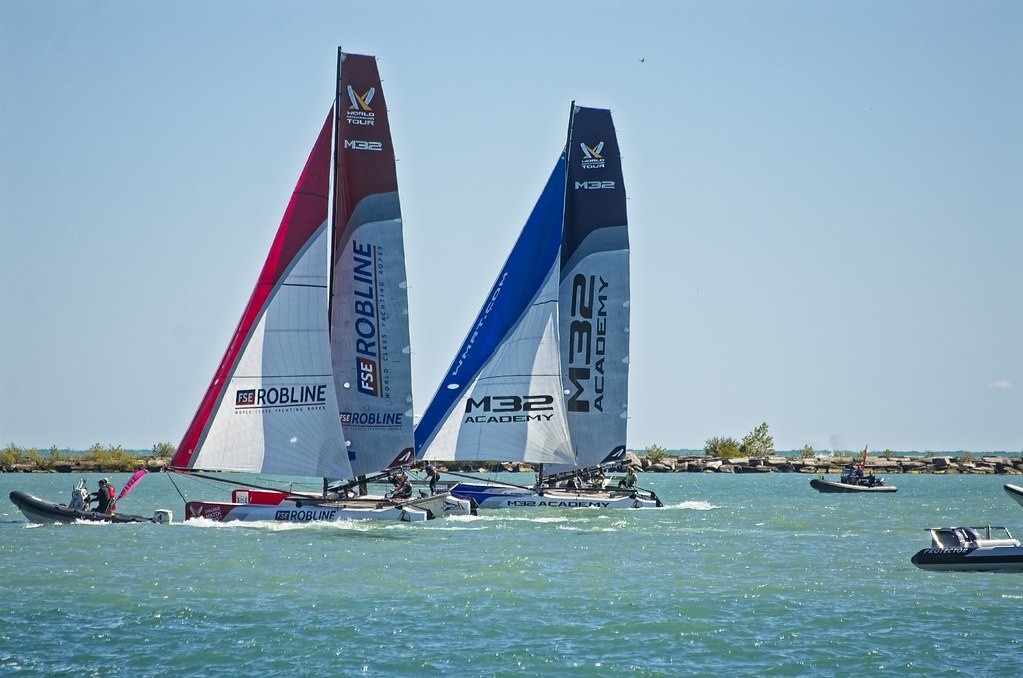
[[599, 474, 603, 478], [97, 480, 105, 484], [103, 478, 108, 483], [628, 467, 634, 472]]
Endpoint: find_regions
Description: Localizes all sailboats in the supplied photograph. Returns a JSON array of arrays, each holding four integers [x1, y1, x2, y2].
[[415, 99, 663, 509], [159, 44, 472, 523]]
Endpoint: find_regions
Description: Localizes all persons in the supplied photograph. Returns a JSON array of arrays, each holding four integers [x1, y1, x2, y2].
[[566, 467, 605, 489], [389, 475, 412, 499], [87, 478, 117, 514], [618, 468, 638, 489], [423, 460, 440, 496]]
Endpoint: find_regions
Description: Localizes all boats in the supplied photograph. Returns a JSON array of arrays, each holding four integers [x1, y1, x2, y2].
[[7, 488, 172, 525], [808, 444, 897, 494], [1003, 483, 1023, 506], [910, 525, 1023, 570]]
